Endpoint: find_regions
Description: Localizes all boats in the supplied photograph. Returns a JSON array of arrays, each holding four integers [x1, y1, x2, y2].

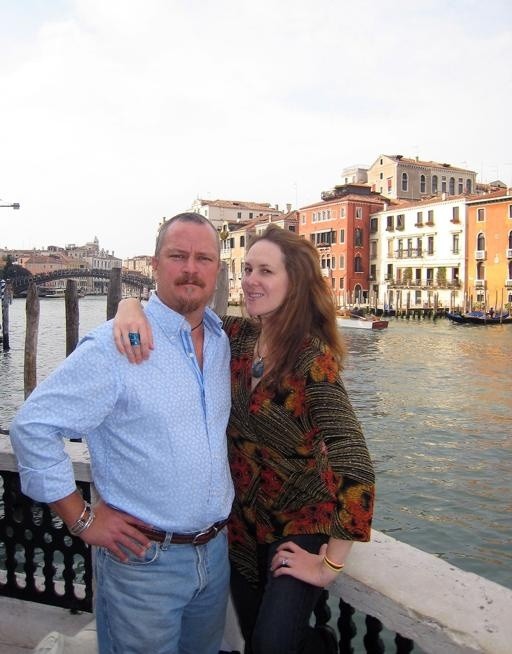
[[444, 308, 510, 323], [45, 288, 81, 298], [458, 312, 511, 324], [333, 312, 388, 332]]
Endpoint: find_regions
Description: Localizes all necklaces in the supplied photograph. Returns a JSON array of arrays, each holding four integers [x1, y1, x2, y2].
[[251, 333, 282, 379], [191, 316, 204, 334]]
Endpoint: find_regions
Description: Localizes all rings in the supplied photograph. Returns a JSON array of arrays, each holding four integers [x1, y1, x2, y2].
[[282, 559, 287, 566], [129, 333, 140, 348]]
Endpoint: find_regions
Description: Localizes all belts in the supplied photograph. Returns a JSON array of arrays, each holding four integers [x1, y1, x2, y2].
[[131, 516, 233, 547]]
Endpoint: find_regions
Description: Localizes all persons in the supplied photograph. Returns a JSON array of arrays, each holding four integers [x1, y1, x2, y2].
[[110, 224, 377, 652], [7, 212, 237, 654], [489, 306, 496, 319]]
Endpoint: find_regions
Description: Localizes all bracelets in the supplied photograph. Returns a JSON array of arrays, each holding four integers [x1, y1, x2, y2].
[[69, 500, 95, 537], [323, 555, 344, 573]]
